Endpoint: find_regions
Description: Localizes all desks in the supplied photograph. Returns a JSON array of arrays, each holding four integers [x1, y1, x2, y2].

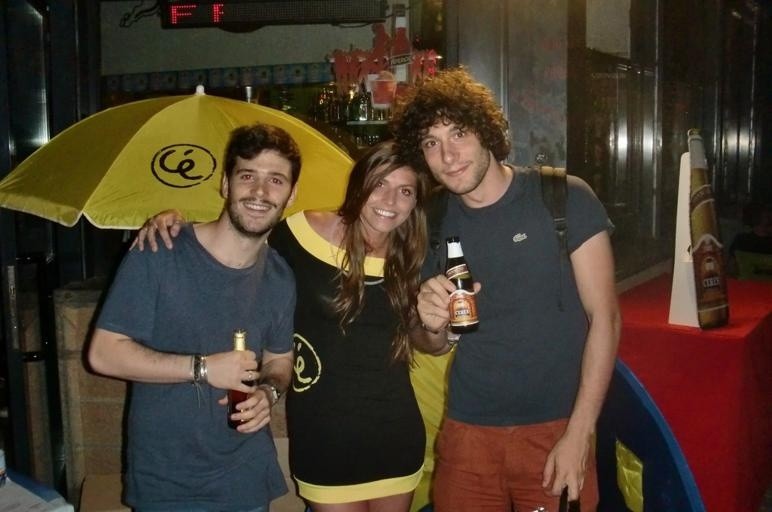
[[610, 272, 772, 511]]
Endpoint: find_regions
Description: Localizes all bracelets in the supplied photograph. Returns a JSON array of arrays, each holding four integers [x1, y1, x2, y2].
[[421, 324, 449, 335], [449, 339, 459, 349], [192, 355, 206, 387]]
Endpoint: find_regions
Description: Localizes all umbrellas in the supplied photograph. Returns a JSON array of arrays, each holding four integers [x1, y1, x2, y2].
[[0, 84, 362, 232]]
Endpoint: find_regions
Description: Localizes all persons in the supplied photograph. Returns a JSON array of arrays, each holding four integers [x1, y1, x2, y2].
[[386, 63, 620, 512], [730, 207, 772, 255], [128, 141, 461, 512]]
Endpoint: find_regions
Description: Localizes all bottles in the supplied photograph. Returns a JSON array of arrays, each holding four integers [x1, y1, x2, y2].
[[444, 236, 480, 334], [226, 328, 255, 430], [313, 82, 394, 125], [687, 126, 730, 329], [388, 16, 412, 86]]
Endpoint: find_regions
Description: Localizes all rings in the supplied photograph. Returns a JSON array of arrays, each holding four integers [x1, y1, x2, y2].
[[249, 371, 252, 382]]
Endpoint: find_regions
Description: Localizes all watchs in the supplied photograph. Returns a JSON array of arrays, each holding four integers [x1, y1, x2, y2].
[[260, 381, 282, 404]]
